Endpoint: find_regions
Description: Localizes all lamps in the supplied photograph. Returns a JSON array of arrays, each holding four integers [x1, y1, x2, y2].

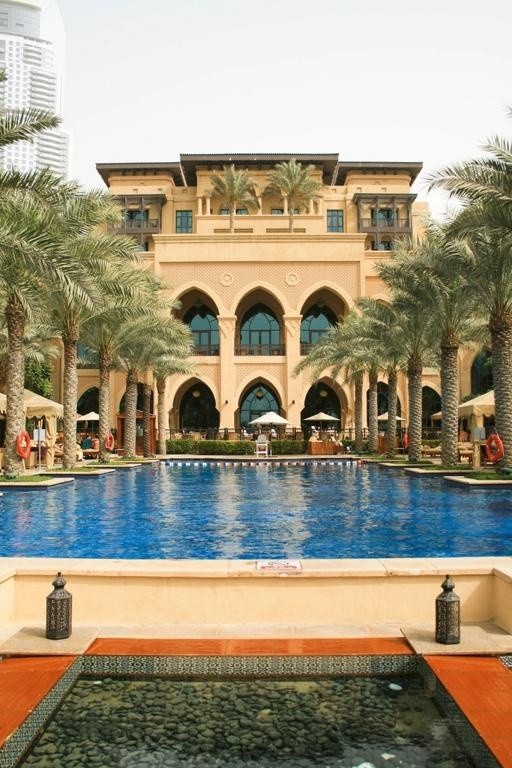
[[434, 574, 460, 643], [46, 572, 72, 640]]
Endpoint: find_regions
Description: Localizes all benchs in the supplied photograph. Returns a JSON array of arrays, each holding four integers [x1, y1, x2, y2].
[[82, 448, 99, 458]]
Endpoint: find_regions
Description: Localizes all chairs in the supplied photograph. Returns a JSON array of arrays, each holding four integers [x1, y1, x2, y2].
[[420, 441, 474, 463], [255, 435, 268, 457]]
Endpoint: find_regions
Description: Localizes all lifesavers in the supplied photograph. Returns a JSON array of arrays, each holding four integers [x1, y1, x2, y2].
[[17, 432, 31, 458], [104, 433, 115, 452], [486, 435, 503, 461], [404, 431, 408, 448]]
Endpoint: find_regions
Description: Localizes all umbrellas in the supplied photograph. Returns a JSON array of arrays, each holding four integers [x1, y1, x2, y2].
[[378, 411, 404, 420], [78, 411, 99, 436], [248, 410, 290, 428], [304, 411, 342, 430]]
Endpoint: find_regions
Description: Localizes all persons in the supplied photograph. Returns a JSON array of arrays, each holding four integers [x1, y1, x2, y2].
[[91, 435, 100, 459], [271, 425, 277, 440], [240, 426, 247, 440], [251, 431, 256, 441], [81, 434, 92, 458]]
